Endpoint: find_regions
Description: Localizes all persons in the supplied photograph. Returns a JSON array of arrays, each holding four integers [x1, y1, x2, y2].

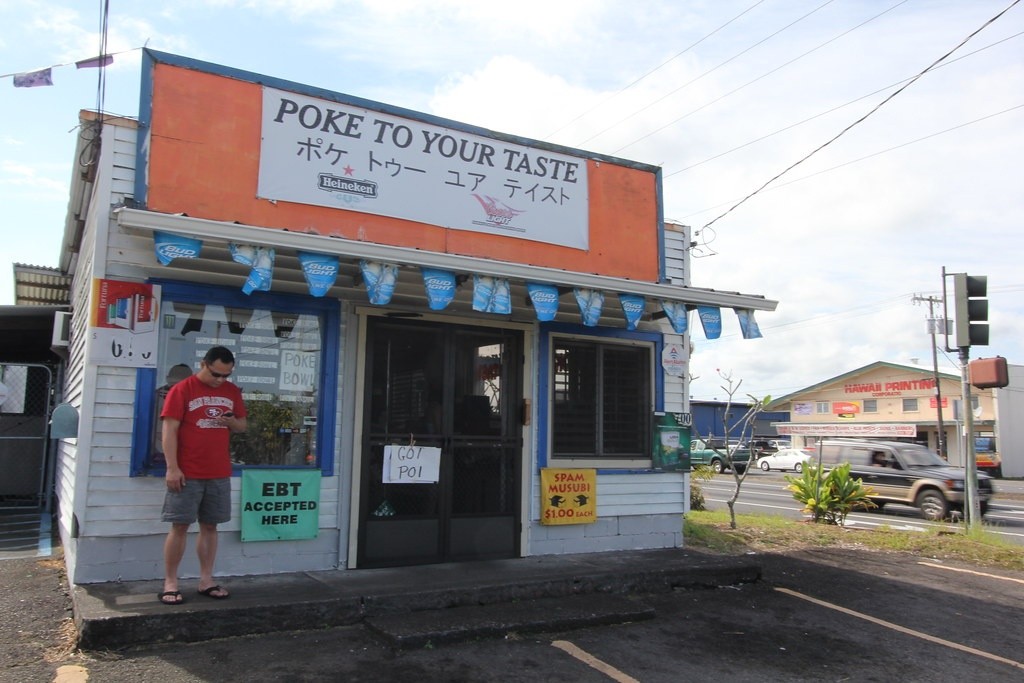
[[157, 346, 247, 605], [871, 451, 893, 469], [149, 364, 193, 465]]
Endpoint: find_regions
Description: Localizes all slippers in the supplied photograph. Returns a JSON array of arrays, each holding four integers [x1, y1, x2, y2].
[[158, 591, 183, 605], [197, 584, 231, 600]]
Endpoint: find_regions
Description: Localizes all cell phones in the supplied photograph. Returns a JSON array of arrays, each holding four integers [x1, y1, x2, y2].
[[221, 412, 235, 417]]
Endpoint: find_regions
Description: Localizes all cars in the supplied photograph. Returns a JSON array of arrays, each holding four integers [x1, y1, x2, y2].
[[726, 439, 791, 460], [757, 449, 814, 473]]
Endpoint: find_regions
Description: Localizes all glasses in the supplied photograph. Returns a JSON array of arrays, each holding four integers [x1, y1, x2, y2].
[[206, 363, 232, 378]]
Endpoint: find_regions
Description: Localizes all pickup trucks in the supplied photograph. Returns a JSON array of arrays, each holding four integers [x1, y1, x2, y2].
[[689, 439, 756, 474]]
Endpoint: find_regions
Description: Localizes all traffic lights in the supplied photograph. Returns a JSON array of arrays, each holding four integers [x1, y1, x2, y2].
[[953, 273, 989, 348]]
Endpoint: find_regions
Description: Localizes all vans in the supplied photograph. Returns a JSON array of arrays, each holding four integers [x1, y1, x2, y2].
[[810, 438, 993, 522]]
